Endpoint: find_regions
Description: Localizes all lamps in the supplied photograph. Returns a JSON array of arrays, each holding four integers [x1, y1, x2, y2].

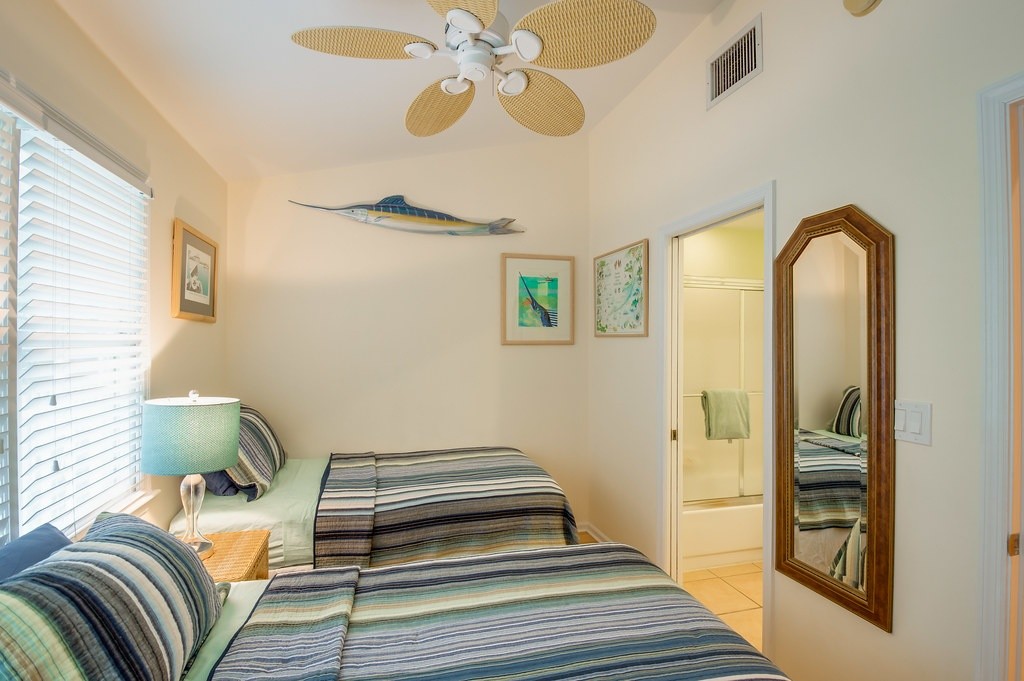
[[140, 389, 241, 559]]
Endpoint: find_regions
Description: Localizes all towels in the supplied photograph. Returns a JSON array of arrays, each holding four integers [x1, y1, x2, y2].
[[701, 389, 752, 441]]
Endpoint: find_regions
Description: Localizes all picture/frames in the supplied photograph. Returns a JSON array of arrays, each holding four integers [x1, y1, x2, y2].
[[171, 217, 220, 324], [593, 239, 648, 336], [500, 253, 575, 346]]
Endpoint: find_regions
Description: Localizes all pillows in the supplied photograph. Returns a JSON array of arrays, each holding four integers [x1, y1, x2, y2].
[[0, 510, 222, 681], [225, 403, 287, 503], [825, 384, 862, 438], [198, 470, 239, 496], [0, 522, 73, 581]]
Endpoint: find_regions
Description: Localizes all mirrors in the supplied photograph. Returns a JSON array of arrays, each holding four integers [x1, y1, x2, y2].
[[774, 204, 895, 633]]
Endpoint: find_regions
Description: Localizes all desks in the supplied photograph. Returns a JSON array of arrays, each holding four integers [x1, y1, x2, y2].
[[177, 529, 270, 584]]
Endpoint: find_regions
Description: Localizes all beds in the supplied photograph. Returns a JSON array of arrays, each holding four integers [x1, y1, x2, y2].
[[792, 430, 863, 531], [168, 447, 579, 578], [178, 540, 789, 681]]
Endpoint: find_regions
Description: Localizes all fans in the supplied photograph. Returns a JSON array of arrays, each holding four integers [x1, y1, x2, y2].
[[291, 0, 656, 137]]
